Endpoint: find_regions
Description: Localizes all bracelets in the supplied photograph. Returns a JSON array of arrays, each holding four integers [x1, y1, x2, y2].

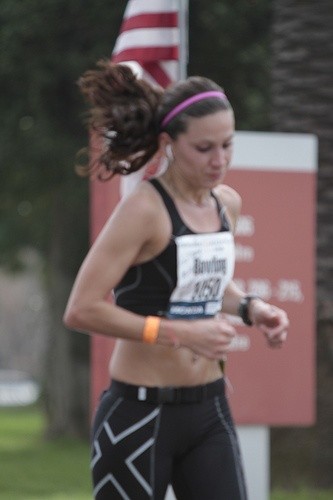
[[143, 315, 160, 344], [238, 295, 257, 326]]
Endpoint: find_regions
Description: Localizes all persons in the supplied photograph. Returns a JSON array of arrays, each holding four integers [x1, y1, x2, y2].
[[63, 58, 289, 500]]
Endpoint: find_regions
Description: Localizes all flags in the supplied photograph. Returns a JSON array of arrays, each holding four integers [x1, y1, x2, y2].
[[108, 0, 188, 203]]
